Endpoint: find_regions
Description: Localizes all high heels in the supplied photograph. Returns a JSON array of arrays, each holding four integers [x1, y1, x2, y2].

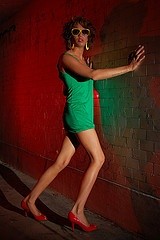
[[67, 211, 97, 233], [20, 200, 48, 221]]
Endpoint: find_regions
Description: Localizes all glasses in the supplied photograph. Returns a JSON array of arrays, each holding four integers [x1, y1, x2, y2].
[[71, 28, 90, 36]]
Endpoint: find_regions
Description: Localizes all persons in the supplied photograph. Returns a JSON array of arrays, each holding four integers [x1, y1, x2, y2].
[[21, 16, 145, 232]]
